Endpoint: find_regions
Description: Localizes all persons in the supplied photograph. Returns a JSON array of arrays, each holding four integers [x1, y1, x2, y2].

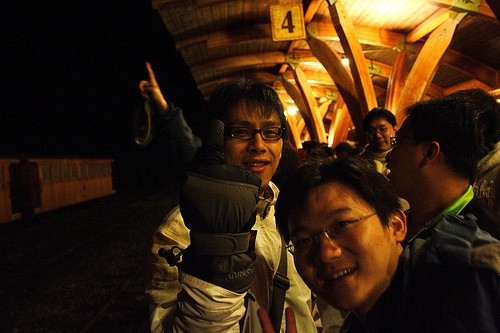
[[139, 62, 357, 204], [148, 80, 323, 333], [274, 160, 500, 333], [385, 89, 500, 231], [353, 108, 399, 173]]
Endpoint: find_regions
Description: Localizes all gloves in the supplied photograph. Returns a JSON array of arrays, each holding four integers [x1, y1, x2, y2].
[[158, 119, 263, 295]]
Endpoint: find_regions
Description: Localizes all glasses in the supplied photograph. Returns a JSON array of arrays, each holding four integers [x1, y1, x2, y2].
[[224, 126, 286, 141], [365, 126, 393, 136], [284, 212, 378, 258], [390, 134, 397, 148]]
